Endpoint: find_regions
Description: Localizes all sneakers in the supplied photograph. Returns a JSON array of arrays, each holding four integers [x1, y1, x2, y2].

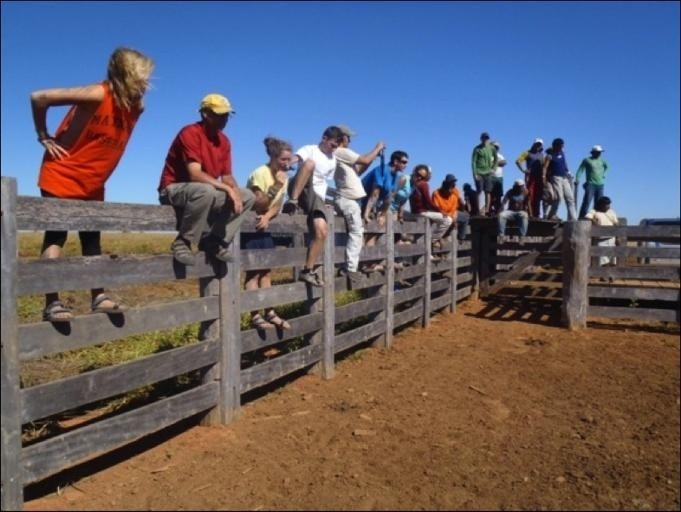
[[283, 199, 298, 212], [198, 237, 233, 262], [394, 262, 402, 270], [363, 262, 383, 272], [299, 270, 324, 286], [169, 239, 195, 265], [340, 268, 367, 280]]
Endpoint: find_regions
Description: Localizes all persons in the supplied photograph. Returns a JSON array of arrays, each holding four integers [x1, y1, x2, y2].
[[584, 196, 619, 283], [376, 164, 431, 272], [470, 131, 498, 215], [541, 138, 577, 223], [241, 136, 293, 332], [29, 47, 153, 322], [156, 94, 254, 266], [333, 126, 385, 285], [462, 183, 477, 217], [410, 165, 453, 252], [497, 180, 532, 246], [280, 126, 343, 287], [574, 145, 608, 220], [361, 150, 408, 271], [430, 174, 469, 248], [514, 138, 548, 221], [489, 142, 506, 215]]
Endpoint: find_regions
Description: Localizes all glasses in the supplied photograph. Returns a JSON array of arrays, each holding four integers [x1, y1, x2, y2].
[[397, 160, 407, 164], [416, 172, 425, 179]]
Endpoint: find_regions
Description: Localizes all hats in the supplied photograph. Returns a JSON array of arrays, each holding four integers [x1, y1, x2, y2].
[[338, 126, 354, 137], [199, 94, 236, 114], [514, 179, 525, 187], [535, 139, 543, 144], [592, 145, 604, 152], [443, 175, 457, 182]]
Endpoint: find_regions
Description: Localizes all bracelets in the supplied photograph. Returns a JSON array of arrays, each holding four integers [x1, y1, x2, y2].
[[37, 137, 53, 142]]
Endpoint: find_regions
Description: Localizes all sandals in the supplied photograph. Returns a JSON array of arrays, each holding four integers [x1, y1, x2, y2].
[[250, 315, 275, 330], [92, 293, 128, 312], [265, 313, 290, 329], [43, 301, 74, 323]]
